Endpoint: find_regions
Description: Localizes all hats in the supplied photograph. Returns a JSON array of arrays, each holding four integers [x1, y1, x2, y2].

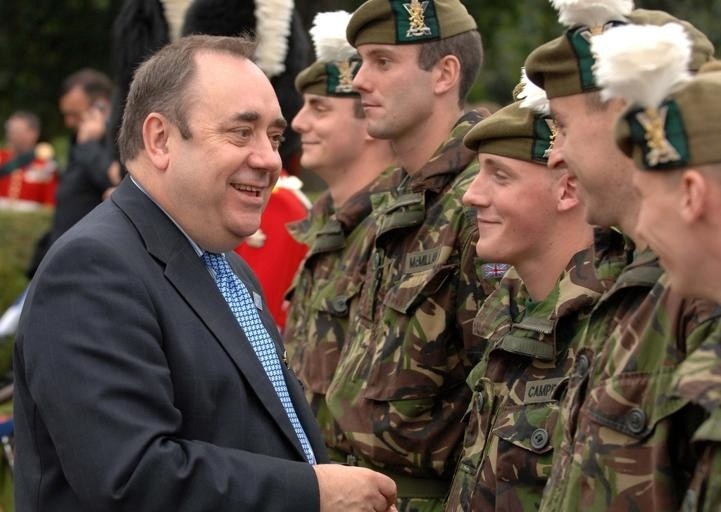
[[464, 82, 556, 167], [520, 10, 720, 170], [346, 1, 478, 46], [296, 57, 361, 97]]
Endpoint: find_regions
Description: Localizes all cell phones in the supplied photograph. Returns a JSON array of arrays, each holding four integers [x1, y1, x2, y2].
[[94, 102, 106, 113]]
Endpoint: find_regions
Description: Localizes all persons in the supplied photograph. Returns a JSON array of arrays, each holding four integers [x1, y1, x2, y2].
[[1, 0, 720, 512]]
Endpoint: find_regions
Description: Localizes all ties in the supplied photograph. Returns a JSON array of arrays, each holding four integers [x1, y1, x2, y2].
[[203, 248, 318, 469]]
